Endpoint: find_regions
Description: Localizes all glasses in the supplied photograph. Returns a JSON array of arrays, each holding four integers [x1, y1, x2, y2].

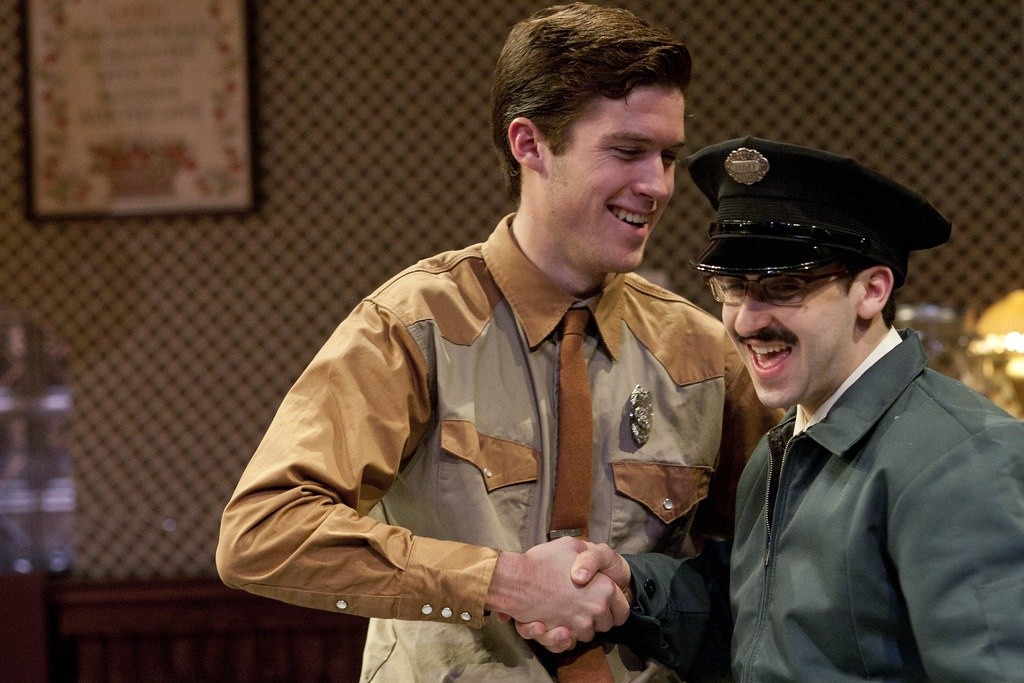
[[702, 269, 859, 306]]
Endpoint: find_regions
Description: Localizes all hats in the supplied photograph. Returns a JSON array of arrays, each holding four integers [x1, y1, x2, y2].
[[684, 135, 952, 289]]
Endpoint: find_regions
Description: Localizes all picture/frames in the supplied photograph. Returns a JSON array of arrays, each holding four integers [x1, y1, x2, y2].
[[17, 0, 260, 222]]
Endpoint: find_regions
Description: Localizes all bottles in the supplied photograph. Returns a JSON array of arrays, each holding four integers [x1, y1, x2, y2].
[[0, 321, 76, 573]]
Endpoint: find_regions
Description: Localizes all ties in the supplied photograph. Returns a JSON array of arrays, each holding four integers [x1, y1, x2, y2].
[[548, 309, 615, 683]]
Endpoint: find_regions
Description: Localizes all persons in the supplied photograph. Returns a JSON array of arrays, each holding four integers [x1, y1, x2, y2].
[[496, 136, 1024, 683], [215, 0, 789, 683]]
